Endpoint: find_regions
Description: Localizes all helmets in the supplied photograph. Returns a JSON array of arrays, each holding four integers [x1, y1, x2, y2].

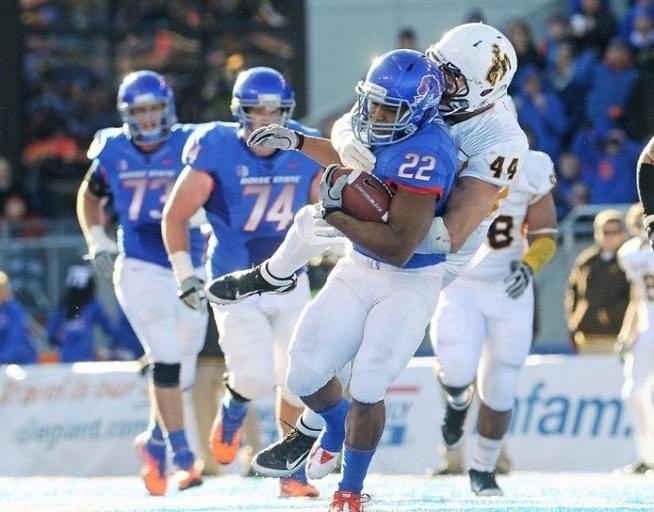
[[426, 25, 518, 116], [230, 67, 295, 135], [350, 48, 443, 146], [119, 71, 177, 146]]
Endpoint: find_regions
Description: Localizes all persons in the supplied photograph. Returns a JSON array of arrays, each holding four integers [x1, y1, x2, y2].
[[161, 66, 328, 498], [428, 149, 561, 498], [285, 48, 460, 511], [501, 0, 653, 356], [203, 22, 529, 477], [76, 71, 217, 497], [0, 0, 307, 364], [616, 202, 654, 475]]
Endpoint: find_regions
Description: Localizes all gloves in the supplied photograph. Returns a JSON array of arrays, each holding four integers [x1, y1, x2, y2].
[[505, 259, 532, 299], [167, 251, 205, 310], [337, 129, 374, 170], [248, 123, 304, 152], [85, 225, 120, 279], [319, 162, 347, 218]]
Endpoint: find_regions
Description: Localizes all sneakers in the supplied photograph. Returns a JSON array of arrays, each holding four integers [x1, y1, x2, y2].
[[443, 382, 475, 450], [209, 399, 246, 464], [468, 469, 502, 497], [134, 430, 168, 495], [205, 260, 298, 305], [251, 428, 361, 512], [173, 450, 202, 490]]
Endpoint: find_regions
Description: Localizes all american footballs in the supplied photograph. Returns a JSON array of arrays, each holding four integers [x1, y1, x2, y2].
[[331, 167, 391, 225]]
